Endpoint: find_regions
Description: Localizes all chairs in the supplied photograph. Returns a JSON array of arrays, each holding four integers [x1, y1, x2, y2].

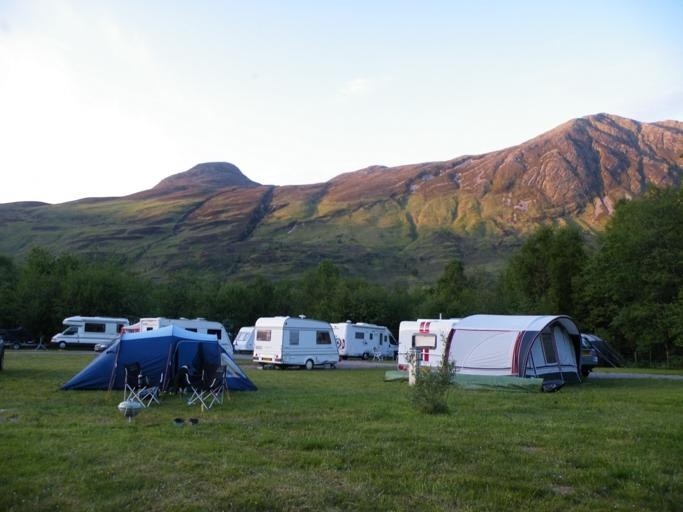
[[186, 364, 226, 410], [123, 362, 162, 408]]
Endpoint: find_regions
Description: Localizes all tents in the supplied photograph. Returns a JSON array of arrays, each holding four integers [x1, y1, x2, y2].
[[443, 314, 582, 391], [60, 324, 258, 392]]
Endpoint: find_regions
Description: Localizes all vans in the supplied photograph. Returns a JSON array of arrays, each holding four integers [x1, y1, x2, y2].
[[232, 326, 255, 352], [331, 322, 398, 359], [0, 328, 48, 350], [50, 314, 128, 348], [140, 317, 234, 363], [254, 317, 339, 369]]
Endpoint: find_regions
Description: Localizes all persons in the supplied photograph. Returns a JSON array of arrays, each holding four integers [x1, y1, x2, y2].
[[174, 365, 191, 393]]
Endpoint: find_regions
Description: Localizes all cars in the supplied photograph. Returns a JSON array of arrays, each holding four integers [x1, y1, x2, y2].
[[579, 334, 599, 376]]
